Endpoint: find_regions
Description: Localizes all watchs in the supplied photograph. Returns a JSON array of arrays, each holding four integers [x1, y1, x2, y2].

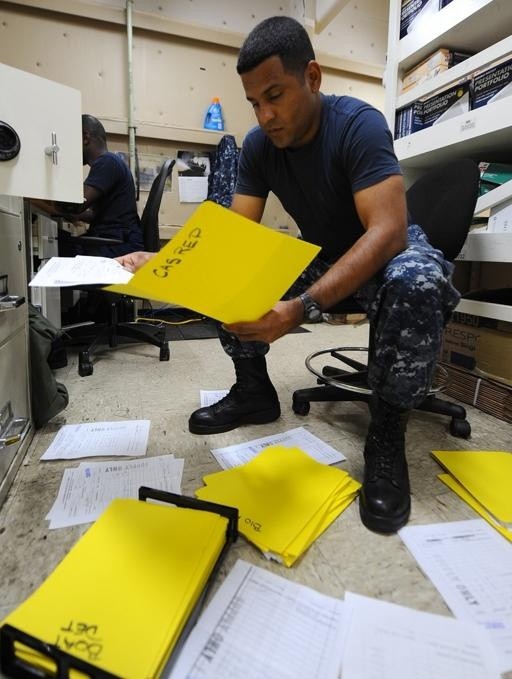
[[299, 292, 324, 322]]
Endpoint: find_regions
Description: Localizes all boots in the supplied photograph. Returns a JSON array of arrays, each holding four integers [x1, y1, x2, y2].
[[358, 399, 413, 535], [188, 355, 282, 435]]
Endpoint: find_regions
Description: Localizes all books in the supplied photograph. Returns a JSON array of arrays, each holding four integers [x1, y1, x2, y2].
[[395, 47, 512, 140], [401, 0, 452, 39]]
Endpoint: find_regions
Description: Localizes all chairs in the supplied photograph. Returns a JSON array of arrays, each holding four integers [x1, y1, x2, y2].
[[288, 157, 486, 439], [46, 155, 178, 376]]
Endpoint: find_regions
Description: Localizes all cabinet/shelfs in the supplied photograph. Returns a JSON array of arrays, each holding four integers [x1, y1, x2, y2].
[[383, 0, 510, 327], [0, 194, 37, 512]]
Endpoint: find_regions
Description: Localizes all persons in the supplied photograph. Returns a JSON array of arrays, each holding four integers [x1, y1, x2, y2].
[[113, 16, 462, 532], [54, 114, 143, 257]]
